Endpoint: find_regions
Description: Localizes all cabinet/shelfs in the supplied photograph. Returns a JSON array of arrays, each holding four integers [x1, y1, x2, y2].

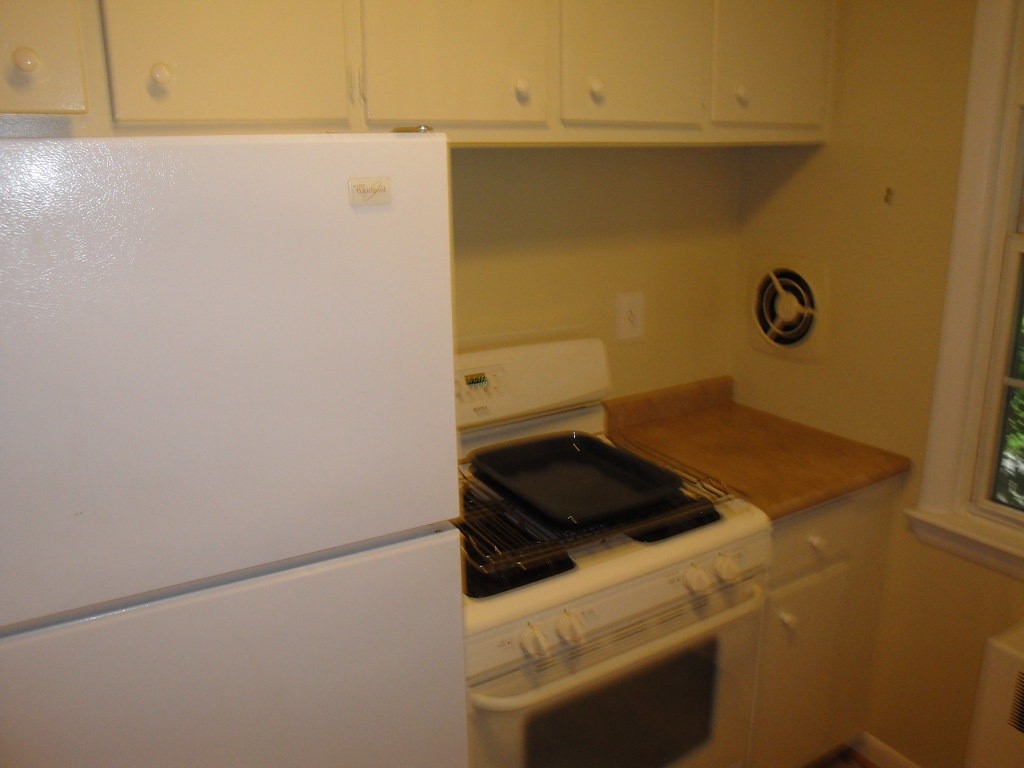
[[744, 473, 900, 768], [0, 0, 835, 149]]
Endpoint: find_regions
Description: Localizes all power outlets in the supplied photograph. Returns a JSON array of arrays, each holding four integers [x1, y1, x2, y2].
[[617, 291, 645, 340]]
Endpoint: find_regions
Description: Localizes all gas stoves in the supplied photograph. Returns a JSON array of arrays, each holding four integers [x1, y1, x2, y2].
[[453, 337, 772, 688]]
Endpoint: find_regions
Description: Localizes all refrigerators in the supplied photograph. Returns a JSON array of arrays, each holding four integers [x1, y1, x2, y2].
[[0, 129, 464, 768]]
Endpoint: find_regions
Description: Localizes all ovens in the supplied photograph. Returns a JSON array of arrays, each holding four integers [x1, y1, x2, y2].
[[465, 575, 767, 768]]
